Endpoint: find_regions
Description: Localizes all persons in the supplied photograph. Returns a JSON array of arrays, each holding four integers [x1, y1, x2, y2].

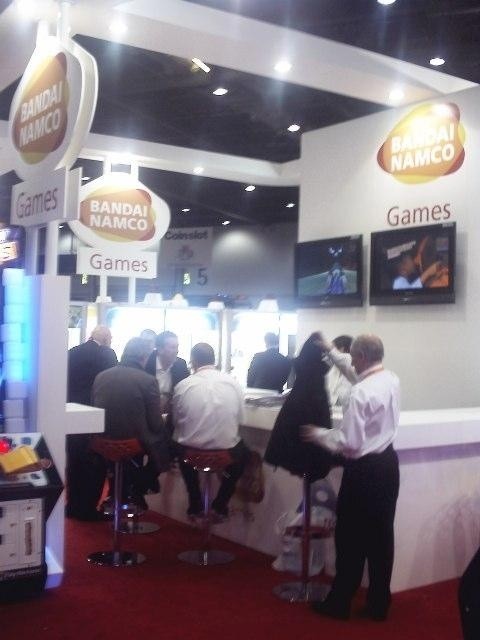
[[246, 332, 289, 391], [393, 254, 441, 290], [322, 257, 349, 294], [65, 327, 117, 521], [146, 332, 191, 406], [325, 334, 353, 405], [91, 339, 170, 508], [302, 331, 404, 624], [141, 326, 156, 355], [171, 342, 250, 519]]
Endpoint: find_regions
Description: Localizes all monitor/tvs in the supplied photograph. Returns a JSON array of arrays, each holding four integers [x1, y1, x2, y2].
[[293, 234, 364, 309], [368, 221, 457, 306]]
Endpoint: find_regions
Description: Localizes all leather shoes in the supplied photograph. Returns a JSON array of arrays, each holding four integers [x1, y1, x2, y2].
[[309, 598, 352, 620], [358, 605, 388, 622]]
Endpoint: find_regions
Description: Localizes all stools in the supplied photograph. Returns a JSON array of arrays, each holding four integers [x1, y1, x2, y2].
[[179, 447, 242, 563], [269, 461, 334, 606], [83, 437, 150, 567]]
